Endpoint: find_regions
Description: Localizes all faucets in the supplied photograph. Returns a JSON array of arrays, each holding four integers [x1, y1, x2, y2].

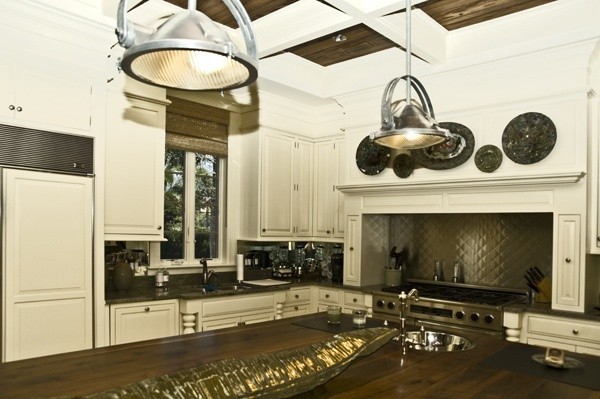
[[199, 256, 215, 286], [398, 287, 421, 354]]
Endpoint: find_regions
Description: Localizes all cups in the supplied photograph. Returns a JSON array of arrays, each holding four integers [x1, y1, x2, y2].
[[352, 306, 368, 328], [326, 305, 342, 324]]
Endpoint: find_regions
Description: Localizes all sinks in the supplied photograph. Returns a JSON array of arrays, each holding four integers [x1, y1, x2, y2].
[[394, 330, 477, 354]]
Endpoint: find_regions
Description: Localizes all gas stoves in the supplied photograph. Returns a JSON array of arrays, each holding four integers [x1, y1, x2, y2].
[[372, 283, 518, 340]]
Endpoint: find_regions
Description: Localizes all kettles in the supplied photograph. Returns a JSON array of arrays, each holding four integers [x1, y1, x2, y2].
[[271, 261, 294, 279]]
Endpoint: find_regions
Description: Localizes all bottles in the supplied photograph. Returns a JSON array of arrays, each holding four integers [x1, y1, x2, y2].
[[277, 245, 285, 260], [331, 245, 342, 256], [314, 245, 325, 260], [296, 244, 304, 264]]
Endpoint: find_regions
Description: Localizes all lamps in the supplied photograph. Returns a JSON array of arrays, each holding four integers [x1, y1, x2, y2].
[[371, 0, 449, 150], [115, 0, 259, 91]]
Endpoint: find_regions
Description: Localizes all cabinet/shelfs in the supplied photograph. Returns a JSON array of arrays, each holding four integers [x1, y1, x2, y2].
[[557, 215, 581, 307], [344, 293, 365, 315], [110, 298, 180, 346], [261, 126, 312, 237], [527, 317, 600, 357], [317, 289, 338, 313], [281, 288, 310, 318], [312, 139, 345, 238], [345, 216, 358, 282], [202, 294, 274, 332], [104, 106, 163, 236]]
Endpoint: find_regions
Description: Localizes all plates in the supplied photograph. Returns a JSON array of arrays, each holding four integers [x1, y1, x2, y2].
[[532, 353, 586, 370]]
